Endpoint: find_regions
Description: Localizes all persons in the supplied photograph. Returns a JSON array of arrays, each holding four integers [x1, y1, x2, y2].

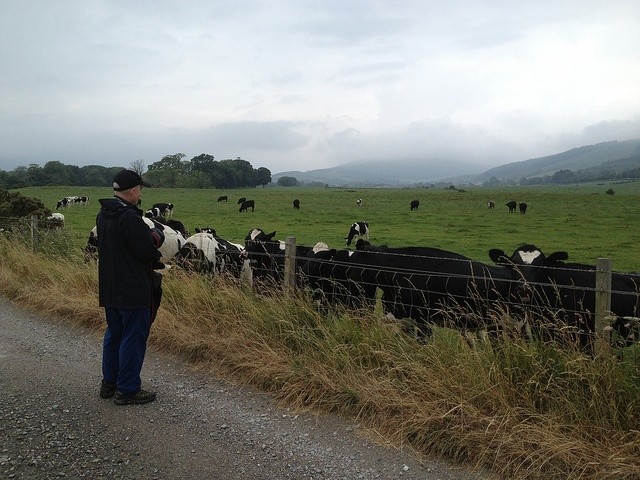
[[96, 170, 165, 405]]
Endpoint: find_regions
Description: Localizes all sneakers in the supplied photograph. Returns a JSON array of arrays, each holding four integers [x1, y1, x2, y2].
[[100, 380, 116, 399], [113, 387, 156, 406]]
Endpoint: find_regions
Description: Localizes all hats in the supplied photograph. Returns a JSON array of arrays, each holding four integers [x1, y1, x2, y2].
[[112, 169, 151, 192]]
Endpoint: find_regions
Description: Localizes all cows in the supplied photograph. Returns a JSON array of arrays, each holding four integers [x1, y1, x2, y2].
[[357, 199, 362, 206], [239, 200, 254, 212], [80, 225, 98, 265], [346, 239, 510, 327], [56, 196, 89, 211], [137, 199, 141, 206], [296, 242, 348, 287], [293, 199, 300, 209], [145, 208, 160, 218], [153, 203, 173, 219], [488, 200, 495, 209], [138, 216, 186, 265], [344, 222, 369, 246], [489, 243, 640, 350], [175, 230, 253, 278], [155, 216, 191, 239], [505, 201, 516, 213], [217, 196, 227, 202], [238, 229, 347, 288], [410, 200, 419, 210], [196, 227, 218, 237], [238, 198, 246, 204], [519, 203, 527, 213]]
